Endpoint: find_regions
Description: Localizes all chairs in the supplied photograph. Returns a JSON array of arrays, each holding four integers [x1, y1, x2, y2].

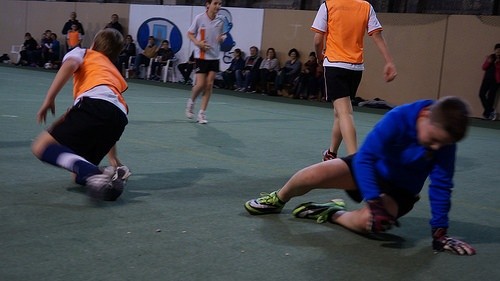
[[122, 50, 177, 83]]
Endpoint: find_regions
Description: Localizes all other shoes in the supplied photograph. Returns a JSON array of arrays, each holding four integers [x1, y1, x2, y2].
[[235, 86, 270, 95], [491, 107, 497, 121], [179, 80, 193, 86], [146, 76, 161, 82]]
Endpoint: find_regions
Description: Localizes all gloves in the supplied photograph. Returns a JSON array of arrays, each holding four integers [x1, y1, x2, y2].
[[429, 227, 476, 256], [366, 196, 400, 234]]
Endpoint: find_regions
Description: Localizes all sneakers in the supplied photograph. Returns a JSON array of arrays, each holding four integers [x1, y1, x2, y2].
[[196, 110, 208, 123], [185, 99, 196, 119], [292, 198, 347, 224], [244, 190, 285, 214], [85, 165, 131, 202], [321, 149, 337, 162]]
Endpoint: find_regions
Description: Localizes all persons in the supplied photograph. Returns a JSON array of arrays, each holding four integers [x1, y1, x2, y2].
[[104, 14, 125, 34], [186, 0, 227, 124], [117, 34, 199, 86], [479, 44, 500, 120], [311, 0, 397, 162], [244, 96, 476, 256], [12, 12, 85, 70], [221, 46, 327, 102], [31, 28, 132, 202]]
